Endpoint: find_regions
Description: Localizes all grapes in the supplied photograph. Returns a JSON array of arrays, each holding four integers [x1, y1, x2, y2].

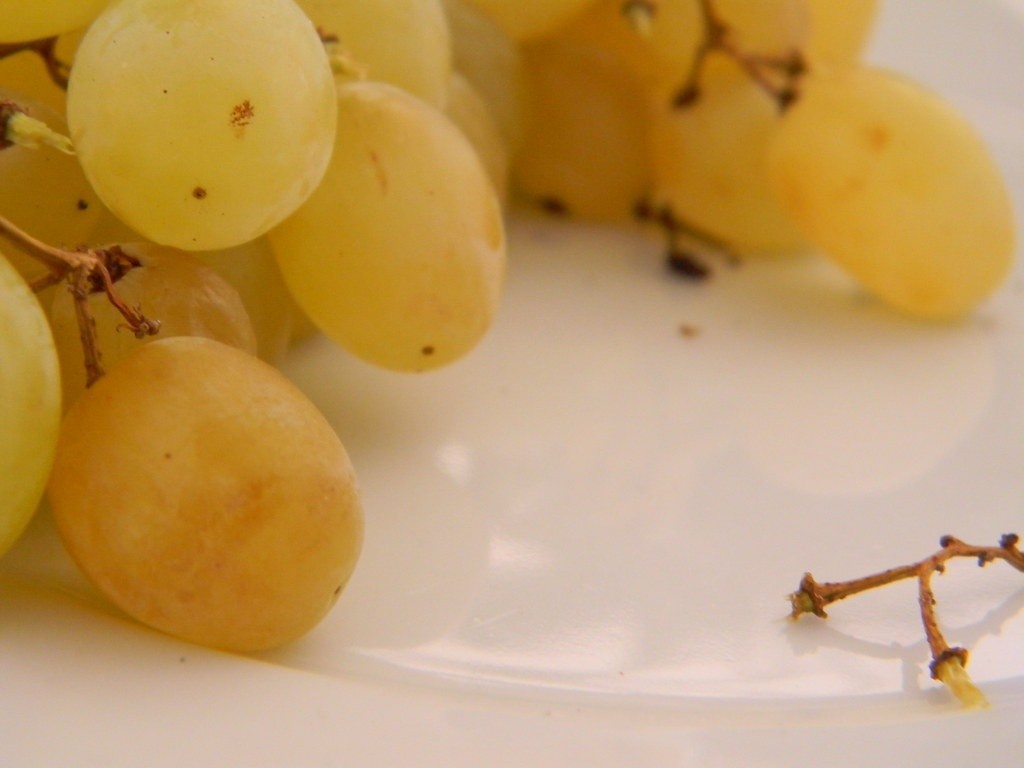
[[447, 0, 1021, 318], [0, 1, 522, 662]]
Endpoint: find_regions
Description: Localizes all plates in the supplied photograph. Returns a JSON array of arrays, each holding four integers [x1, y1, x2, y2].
[[0, 0, 1023, 701]]
